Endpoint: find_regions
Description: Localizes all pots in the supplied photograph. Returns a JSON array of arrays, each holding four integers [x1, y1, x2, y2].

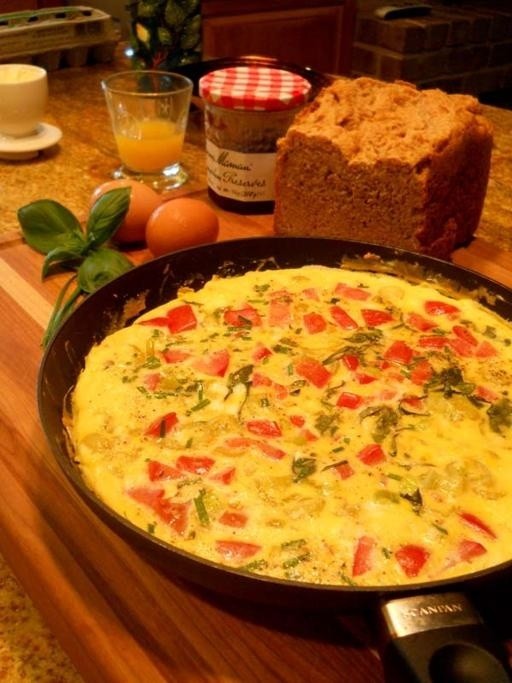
[[169, 49, 334, 119], [36, 235, 512, 683]]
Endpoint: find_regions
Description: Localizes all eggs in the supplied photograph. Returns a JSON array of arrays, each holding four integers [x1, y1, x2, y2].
[[146, 198, 220, 257], [92, 178, 161, 246]]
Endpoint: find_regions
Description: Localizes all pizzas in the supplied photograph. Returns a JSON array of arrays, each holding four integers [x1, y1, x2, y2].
[[68, 264, 512, 587]]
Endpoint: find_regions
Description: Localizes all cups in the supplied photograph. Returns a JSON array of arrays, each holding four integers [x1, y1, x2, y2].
[[100, 69, 194, 193], [0, 63, 49, 137]]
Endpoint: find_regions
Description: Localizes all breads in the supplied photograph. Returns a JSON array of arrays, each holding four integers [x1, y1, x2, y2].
[[272, 75, 493, 258]]
